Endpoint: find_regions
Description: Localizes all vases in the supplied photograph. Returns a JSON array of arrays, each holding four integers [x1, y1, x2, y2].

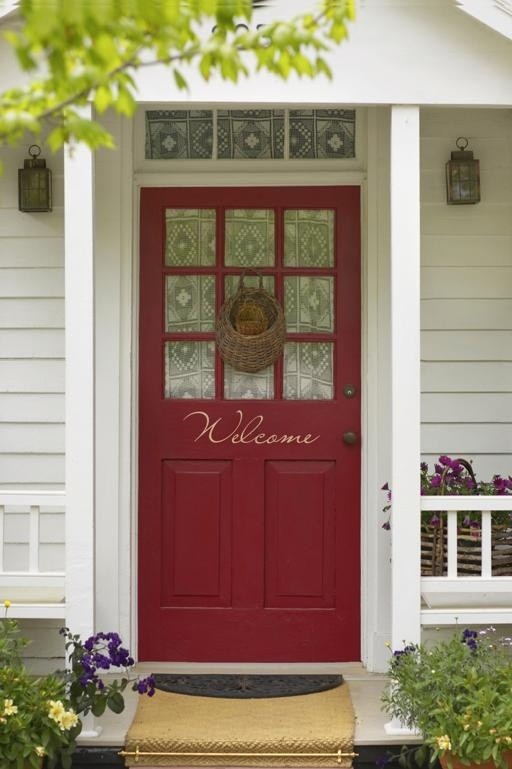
[[438, 747, 511, 769]]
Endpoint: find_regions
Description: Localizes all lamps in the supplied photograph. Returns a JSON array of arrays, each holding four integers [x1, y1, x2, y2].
[[444, 134, 481, 208], [17, 145, 53, 216]]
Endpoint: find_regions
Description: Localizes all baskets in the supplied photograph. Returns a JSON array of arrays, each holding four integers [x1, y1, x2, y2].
[[216, 286, 285, 374]]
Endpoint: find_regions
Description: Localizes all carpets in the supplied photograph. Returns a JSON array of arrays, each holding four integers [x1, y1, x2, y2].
[[147, 672, 345, 701]]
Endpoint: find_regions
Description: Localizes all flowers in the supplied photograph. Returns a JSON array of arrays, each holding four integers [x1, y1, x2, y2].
[[380, 450, 512, 540], [0, 601, 157, 767], [372, 616, 511, 769]]
